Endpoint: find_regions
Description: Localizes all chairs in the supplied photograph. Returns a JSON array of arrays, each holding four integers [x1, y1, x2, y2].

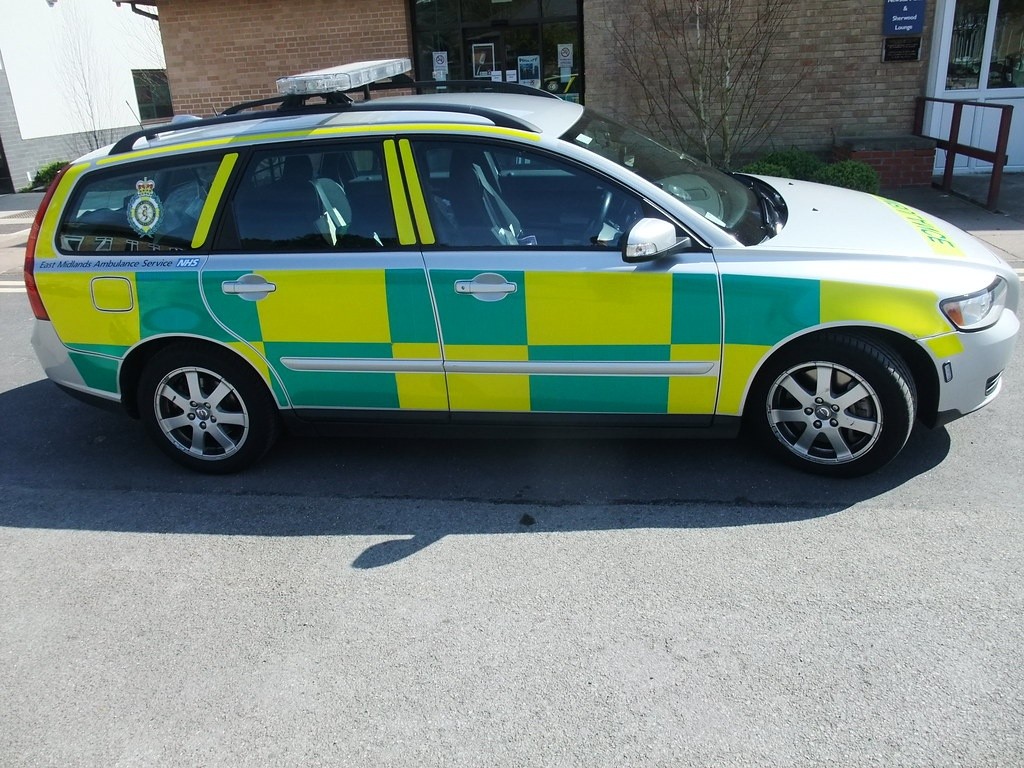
[[379, 151, 466, 247], [268, 156, 353, 246], [448, 148, 526, 241]]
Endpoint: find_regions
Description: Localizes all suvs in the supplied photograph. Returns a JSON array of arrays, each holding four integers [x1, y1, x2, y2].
[[22, 55, 1023, 481]]
